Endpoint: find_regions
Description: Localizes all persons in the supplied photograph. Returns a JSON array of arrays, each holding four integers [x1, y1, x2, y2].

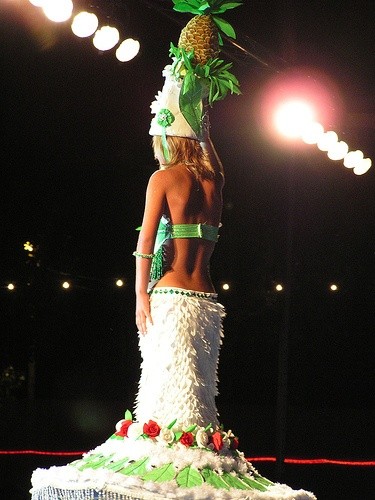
[[29, 66, 318, 500]]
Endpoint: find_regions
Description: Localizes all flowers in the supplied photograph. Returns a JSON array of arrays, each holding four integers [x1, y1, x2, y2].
[[112, 410, 239, 454]]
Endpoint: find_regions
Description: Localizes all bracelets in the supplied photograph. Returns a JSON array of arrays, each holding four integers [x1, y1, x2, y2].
[[132, 251, 156, 259]]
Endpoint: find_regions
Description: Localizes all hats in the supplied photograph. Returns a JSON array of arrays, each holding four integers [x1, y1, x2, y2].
[[148, 75, 202, 141]]
